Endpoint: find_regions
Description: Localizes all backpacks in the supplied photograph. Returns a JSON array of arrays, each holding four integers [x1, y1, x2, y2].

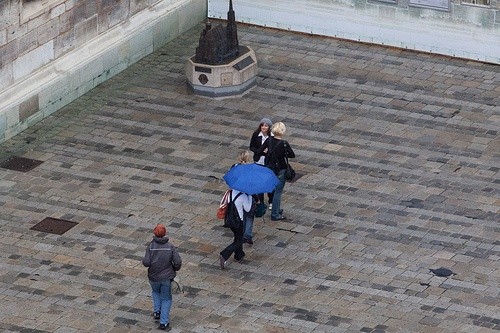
[[223, 190, 243, 229], [265, 137, 283, 175]]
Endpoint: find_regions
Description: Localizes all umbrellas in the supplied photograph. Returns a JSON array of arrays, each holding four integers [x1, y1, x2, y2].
[[223, 163, 280, 195]]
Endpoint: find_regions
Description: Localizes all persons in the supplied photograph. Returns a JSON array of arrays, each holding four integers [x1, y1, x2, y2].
[[230, 150, 261, 244], [250, 118, 274, 210], [219, 189, 252, 269], [142, 224, 182, 329], [258, 121, 295, 221]]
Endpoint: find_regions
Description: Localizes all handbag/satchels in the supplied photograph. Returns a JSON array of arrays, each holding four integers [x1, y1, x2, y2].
[[254, 202, 267, 217], [284, 162, 296, 180], [171, 276, 184, 295], [216, 190, 231, 220]]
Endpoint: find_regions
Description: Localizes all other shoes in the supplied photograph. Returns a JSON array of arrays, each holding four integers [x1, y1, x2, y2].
[[159, 323, 171, 330], [234, 255, 246, 262], [269, 203, 272, 210], [279, 208, 283, 214], [243, 236, 253, 244], [153, 311, 160, 319], [218, 254, 225, 270], [271, 215, 286, 220]]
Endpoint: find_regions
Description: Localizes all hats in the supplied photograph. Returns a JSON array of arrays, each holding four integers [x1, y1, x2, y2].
[[154, 224, 166, 236], [260, 118, 272, 126]]
